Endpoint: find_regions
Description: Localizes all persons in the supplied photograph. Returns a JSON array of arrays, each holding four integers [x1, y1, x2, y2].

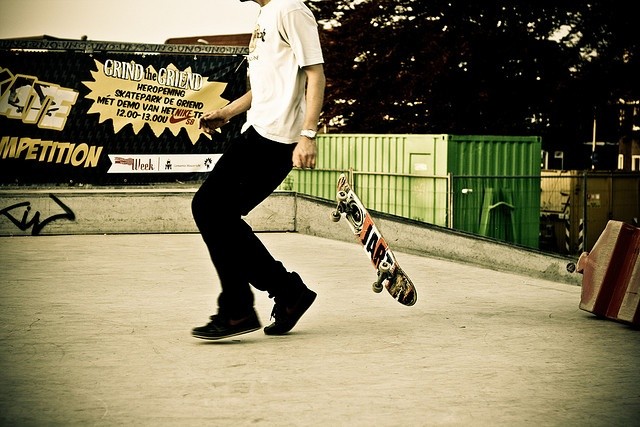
[[190, 0, 327, 340]]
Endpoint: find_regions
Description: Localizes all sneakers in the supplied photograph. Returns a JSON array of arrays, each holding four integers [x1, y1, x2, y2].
[[264, 272, 317, 335], [192, 308, 261, 340]]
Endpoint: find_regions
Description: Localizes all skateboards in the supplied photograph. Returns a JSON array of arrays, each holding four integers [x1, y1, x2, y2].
[[331, 174, 417, 306]]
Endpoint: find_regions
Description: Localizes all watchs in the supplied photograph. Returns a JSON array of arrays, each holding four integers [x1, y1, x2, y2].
[[300, 127, 317, 139]]
